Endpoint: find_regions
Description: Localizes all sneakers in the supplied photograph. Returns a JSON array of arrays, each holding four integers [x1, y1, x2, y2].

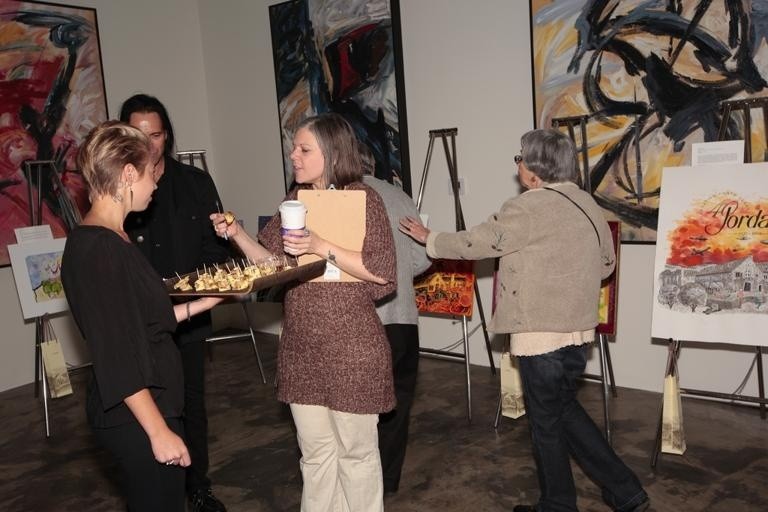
[[187, 486, 228, 511], [512, 504, 578, 511]]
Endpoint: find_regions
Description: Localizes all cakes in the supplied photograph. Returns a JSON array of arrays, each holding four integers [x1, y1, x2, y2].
[[174, 265, 291, 291]]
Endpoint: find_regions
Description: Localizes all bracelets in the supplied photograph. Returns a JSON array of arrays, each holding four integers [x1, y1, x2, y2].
[[186, 299, 191, 323]]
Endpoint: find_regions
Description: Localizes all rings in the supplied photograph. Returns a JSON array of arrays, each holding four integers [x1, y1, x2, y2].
[[213, 224, 219, 232], [166, 459, 175, 468], [305, 229, 311, 238]]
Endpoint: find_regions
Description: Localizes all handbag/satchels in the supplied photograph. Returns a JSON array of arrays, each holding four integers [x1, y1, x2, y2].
[[39, 318, 75, 402], [499, 334, 526, 421], [660, 351, 687, 456]]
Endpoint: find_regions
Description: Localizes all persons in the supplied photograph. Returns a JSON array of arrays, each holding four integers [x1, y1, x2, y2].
[[57, 118, 197, 511], [398, 126, 652, 512], [346, 119, 435, 500], [120, 94, 244, 511], [207, 111, 399, 511]]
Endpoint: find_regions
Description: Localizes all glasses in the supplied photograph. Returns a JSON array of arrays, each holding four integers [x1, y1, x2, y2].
[[514, 155, 523, 164]]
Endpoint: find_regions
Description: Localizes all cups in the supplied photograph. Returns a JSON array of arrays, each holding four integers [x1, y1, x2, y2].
[[279, 200, 306, 238]]
